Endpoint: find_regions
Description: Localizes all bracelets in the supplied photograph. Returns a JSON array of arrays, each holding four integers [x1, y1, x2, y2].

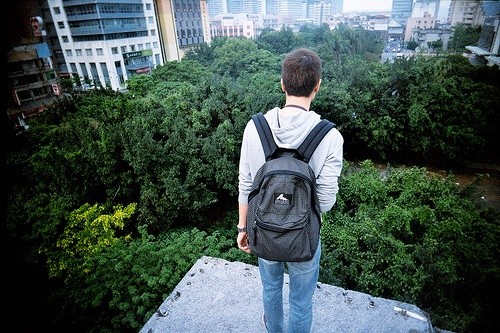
[[237, 225, 247, 232]]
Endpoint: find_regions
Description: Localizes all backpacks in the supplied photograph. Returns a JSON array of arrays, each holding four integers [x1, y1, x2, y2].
[[246, 111, 337, 262]]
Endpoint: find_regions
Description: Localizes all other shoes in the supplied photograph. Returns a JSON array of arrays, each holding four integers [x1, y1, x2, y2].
[[261, 311, 270, 332]]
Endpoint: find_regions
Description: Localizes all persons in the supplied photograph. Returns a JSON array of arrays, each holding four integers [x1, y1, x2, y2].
[[237, 48, 344, 333]]
